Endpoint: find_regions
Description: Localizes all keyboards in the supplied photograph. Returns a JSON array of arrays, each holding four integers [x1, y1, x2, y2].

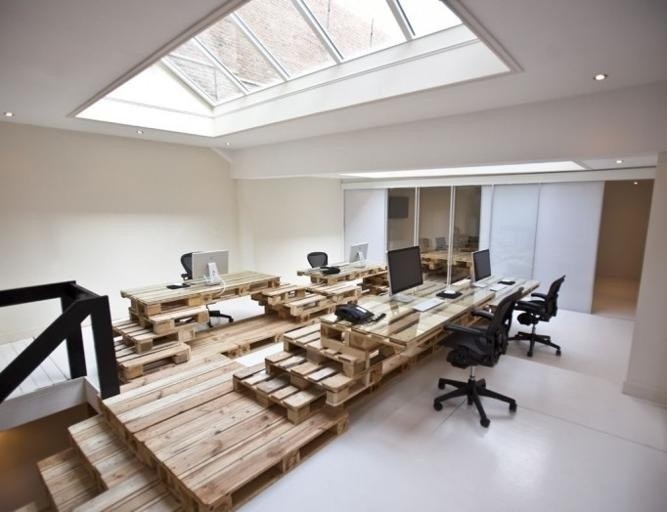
[[183, 278, 204, 285], [489, 278, 512, 292], [335, 262, 351, 267], [413, 295, 444, 312]]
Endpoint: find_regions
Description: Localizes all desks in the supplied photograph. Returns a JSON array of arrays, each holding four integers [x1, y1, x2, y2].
[[322, 281, 495, 353], [473, 274, 540, 306], [297, 263, 380, 283], [120, 270, 279, 315]]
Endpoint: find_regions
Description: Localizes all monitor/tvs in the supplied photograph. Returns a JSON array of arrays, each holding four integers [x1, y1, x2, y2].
[[385, 244, 424, 303], [470, 248, 494, 288], [349, 241, 370, 270], [191, 249, 231, 287], [387, 196, 410, 219]]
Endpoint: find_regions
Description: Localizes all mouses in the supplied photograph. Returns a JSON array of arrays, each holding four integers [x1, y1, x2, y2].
[[183, 280, 192, 289], [444, 289, 455, 294]]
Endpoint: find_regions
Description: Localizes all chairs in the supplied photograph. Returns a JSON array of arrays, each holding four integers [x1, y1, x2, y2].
[[511, 274, 567, 357], [181, 250, 233, 329], [433, 291, 517, 429], [307, 251, 328, 268]]
